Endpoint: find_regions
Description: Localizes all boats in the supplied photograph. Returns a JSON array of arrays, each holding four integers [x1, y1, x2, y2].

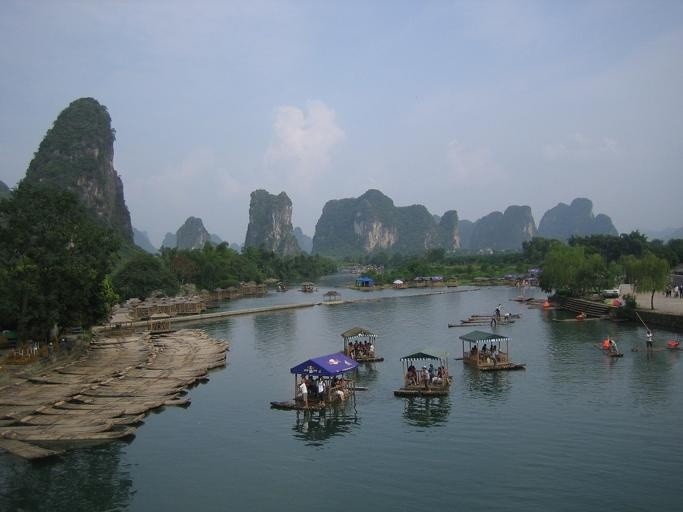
[[631, 345, 683, 352], [462, 330, 526, 370], [270, 352, 359, 411], [298, 281, 319, 292], [526, 298, 561, 310], [276, 282, 288, 292], [394, 348, 453, 395], [0, 329, 229, 460], [594, 342, 623, 357], [552, 317, 602, 322], [340, 327, 384, 364], [448, 313, 520, 327]]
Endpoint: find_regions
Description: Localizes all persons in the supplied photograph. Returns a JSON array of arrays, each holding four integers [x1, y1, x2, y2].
[[666, 283, 683, 298], [471, 344, 498, 365], [490, 304, 512, 326], [298, 375, 345, 408], [647, 331, 654, 348], [609, 340, 618, 352], [348, 340, 374, 359], [613, 294, 632, 306], [406, 364, 446, 392]]
